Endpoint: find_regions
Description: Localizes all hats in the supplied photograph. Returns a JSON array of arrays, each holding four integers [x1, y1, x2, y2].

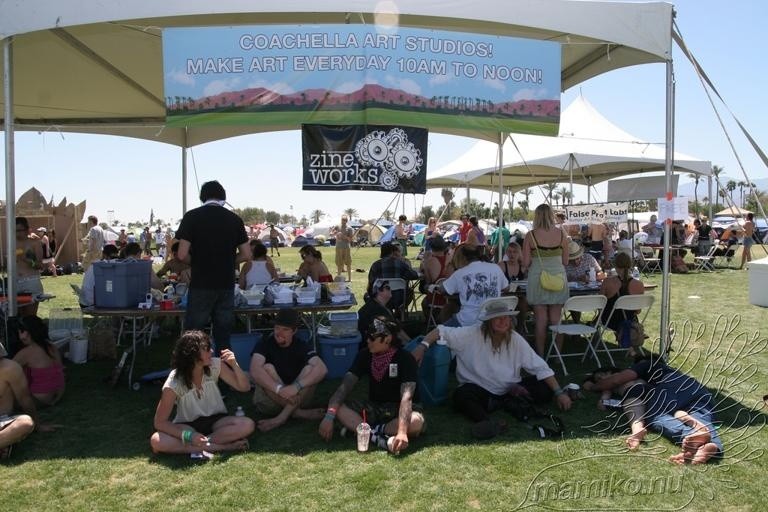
[[36, 226, 47, 233], [567, 241, 585, 260], [478, 295, 520, 322], [372, 278, 390, 295], [271, 308, 302, 326]]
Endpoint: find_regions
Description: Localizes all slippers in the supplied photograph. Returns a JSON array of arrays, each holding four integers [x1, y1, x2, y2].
[[237, 437, 251, 451]]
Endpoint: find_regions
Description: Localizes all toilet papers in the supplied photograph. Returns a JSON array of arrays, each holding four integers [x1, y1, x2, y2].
[[305, 276, 322, 299]]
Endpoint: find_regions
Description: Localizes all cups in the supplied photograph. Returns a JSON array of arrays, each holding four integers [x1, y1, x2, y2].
[[568, 383, 579, 402], [357, 423, 371, 452]]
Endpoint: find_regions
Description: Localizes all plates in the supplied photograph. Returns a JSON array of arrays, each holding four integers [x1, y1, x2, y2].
[[241, 290, 266, 300], [512, 280, 527, 290]]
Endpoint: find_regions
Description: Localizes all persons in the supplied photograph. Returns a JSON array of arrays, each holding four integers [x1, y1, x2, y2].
[[0, 180, 757, 466]]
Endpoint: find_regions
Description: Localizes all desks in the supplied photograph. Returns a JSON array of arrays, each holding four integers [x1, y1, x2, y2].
[[83, 304, 350, 391], [501, 281, 657, 343]]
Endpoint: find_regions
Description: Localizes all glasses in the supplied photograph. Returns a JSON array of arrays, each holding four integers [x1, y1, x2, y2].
[[199, 345, 211, 352], [364, 333, 389, 342], [16, 228, 27, 232]]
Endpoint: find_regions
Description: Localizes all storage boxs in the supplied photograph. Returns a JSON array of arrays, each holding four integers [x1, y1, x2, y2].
[[317, 333, 362, 380], [92, 259, 153, 309], [229, 332, 262, 370], [329, 312, 359, 334]]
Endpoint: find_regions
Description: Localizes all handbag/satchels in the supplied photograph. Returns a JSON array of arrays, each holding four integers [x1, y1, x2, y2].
[[614, 319, 644, 348], [539, 269, 564, 292], [92, 320, 118, 362]]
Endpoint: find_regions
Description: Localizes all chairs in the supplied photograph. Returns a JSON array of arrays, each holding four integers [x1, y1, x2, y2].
[[546, 295, 607, 376], [426, 278, 447, 330], [591, 295, 654, 367], [373, 278, 407, 322], [618, 243, 739, 278]]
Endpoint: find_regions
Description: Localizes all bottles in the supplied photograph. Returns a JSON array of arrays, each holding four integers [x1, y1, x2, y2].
[[236, 407, 244, 416], [602, 399, 622, 407], [160, 272, 178, 310], [586, 266, 640, 282]]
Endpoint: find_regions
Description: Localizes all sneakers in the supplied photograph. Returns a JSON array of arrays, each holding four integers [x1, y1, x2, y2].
[[340, 426, 353, 437], [386, 435, 401, 457]]
